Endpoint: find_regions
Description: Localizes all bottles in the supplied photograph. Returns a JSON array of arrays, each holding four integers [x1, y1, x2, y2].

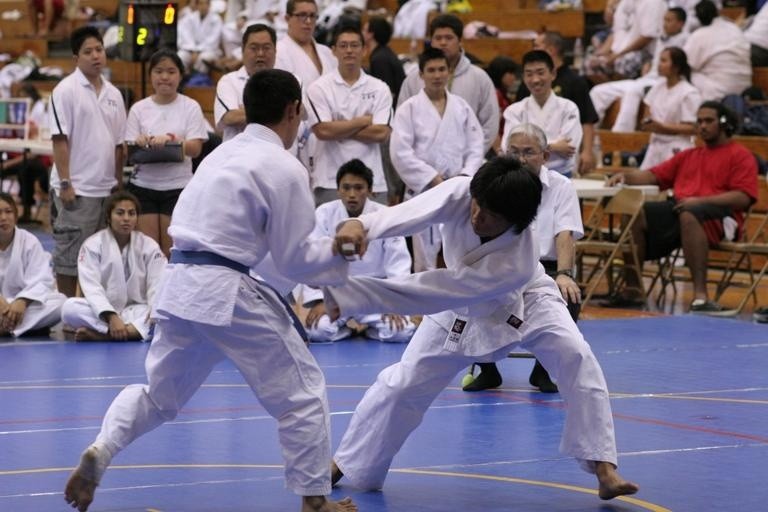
[[592, 135, 603, 169], [573, 37, 583, 77]]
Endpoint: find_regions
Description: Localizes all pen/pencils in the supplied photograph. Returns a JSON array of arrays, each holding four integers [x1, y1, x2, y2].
[[604, 174, 609, 182]]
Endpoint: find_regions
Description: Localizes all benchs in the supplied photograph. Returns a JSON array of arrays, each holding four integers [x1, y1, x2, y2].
[[0, 0, 768, 273]]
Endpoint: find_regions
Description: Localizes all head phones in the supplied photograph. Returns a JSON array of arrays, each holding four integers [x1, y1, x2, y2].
[[720, 106, 728, 126]]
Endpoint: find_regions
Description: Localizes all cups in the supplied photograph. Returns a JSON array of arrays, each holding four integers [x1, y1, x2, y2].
[[612, 151, 621, 167], [16, 104, 24, 124]]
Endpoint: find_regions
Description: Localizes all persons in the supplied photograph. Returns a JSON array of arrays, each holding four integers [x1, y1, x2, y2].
[[306, 158, 639, 501], [1, 2, 768, 347], [463, 125, 582, 394], [57, 71, 353, 512]]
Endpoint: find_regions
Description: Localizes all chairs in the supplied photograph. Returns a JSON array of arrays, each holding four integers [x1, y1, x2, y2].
[[556, 171, 768, 322]]
